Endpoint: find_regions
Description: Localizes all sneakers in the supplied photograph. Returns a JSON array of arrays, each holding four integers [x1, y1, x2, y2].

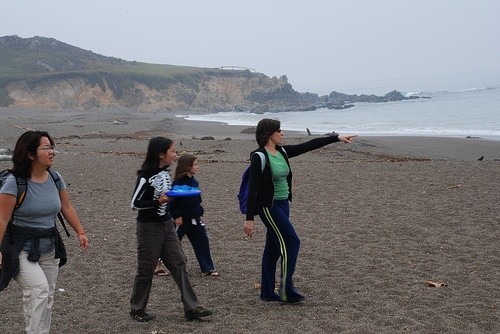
[[130, 309, 155, 322], [184, 305, 215, 320]]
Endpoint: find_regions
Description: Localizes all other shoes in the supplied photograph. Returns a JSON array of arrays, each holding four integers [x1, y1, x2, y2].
[[260, 292, 280, 301], [278, 291, 305, 305]]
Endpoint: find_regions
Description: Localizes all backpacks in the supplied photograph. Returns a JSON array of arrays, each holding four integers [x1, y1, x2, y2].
[[237, 146, 288, 215], [0, 167, 60, 223]]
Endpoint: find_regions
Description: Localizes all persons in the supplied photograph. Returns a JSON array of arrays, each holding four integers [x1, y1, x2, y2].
[[130, 137, 213, 322], [154, 152, 219, 277], [243, 118, 358, 305], [0, 130, 89, 334]]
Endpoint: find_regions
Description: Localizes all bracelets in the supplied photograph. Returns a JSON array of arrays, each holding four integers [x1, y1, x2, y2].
[[77, 233, 85, 236]]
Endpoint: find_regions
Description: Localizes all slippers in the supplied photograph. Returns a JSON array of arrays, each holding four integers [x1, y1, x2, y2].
[[153, 269, 170, 276], [202, 271, 220, 276]]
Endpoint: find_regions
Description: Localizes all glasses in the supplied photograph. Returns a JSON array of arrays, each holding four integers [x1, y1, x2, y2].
[[36, 145, 55, 152]]
